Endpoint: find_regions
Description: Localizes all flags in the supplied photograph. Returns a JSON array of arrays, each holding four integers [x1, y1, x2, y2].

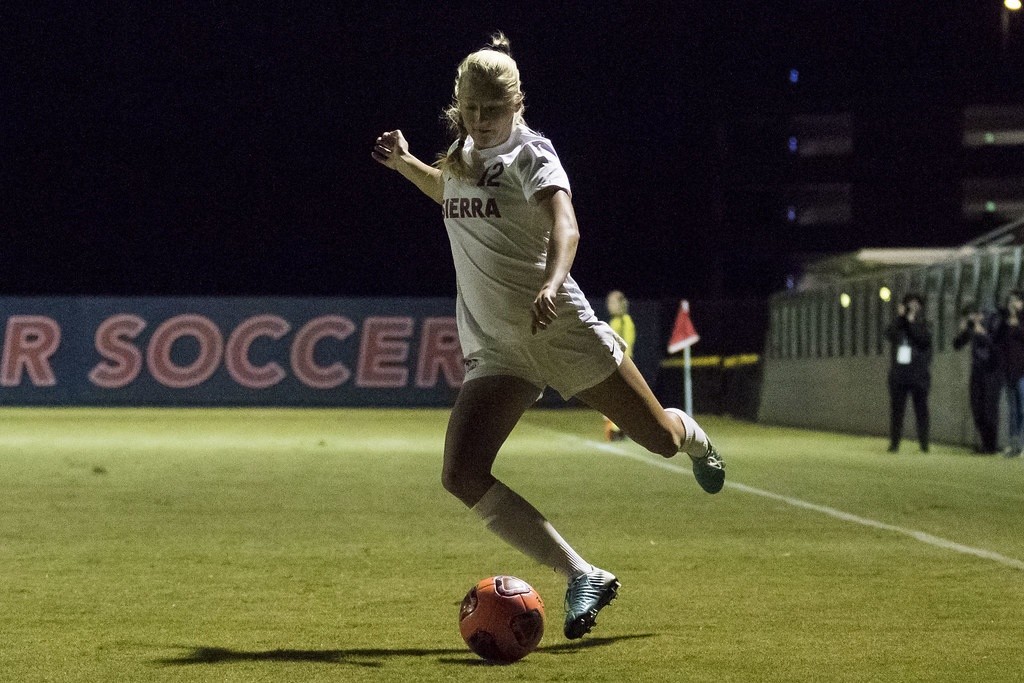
[[668, 308, 699, 353]]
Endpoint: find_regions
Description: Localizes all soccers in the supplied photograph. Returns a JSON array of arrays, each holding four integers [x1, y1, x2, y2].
[[458, 574, 545, 665]]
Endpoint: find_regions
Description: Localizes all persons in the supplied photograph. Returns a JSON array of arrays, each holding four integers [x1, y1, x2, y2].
[[994, 288, 1024, 457], [884, 291, 934, 454], [603, 289, 636, 441], [371, 29, 728, 641], [952, 302, 1001, 456]]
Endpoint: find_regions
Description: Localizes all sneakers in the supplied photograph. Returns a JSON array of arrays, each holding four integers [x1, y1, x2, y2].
[[563, 565, 621, 640], [685, 433, 727, 494]]
